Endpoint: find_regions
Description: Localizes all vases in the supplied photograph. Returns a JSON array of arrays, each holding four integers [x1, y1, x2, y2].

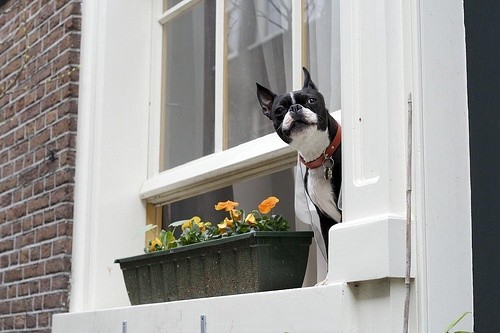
[[115, 232, 315, 306]]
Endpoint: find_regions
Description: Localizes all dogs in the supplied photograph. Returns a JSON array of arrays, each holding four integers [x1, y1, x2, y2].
[[256, 66, 342, 273]]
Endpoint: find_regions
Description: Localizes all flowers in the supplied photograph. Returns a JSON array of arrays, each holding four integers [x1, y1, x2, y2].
[[143, 195, 287, 253]]
